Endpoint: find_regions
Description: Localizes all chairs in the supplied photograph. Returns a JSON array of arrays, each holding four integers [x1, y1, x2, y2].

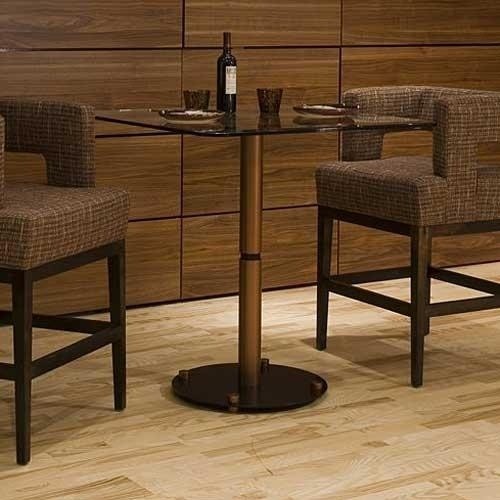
[[315, 86, 500, 387], [1, 98, 132, 465]]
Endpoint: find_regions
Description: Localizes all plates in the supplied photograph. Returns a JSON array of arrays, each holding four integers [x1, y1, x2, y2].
[[292, 103, 359, 117], [157, 110, 225, 122]]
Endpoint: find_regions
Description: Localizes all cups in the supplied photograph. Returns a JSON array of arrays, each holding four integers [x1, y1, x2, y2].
[[257, 87, 283, 114], [183, 89, 210, 110]]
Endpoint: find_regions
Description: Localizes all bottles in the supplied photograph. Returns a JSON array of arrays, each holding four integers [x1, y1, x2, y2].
[[216, 31, 236, 115]]
